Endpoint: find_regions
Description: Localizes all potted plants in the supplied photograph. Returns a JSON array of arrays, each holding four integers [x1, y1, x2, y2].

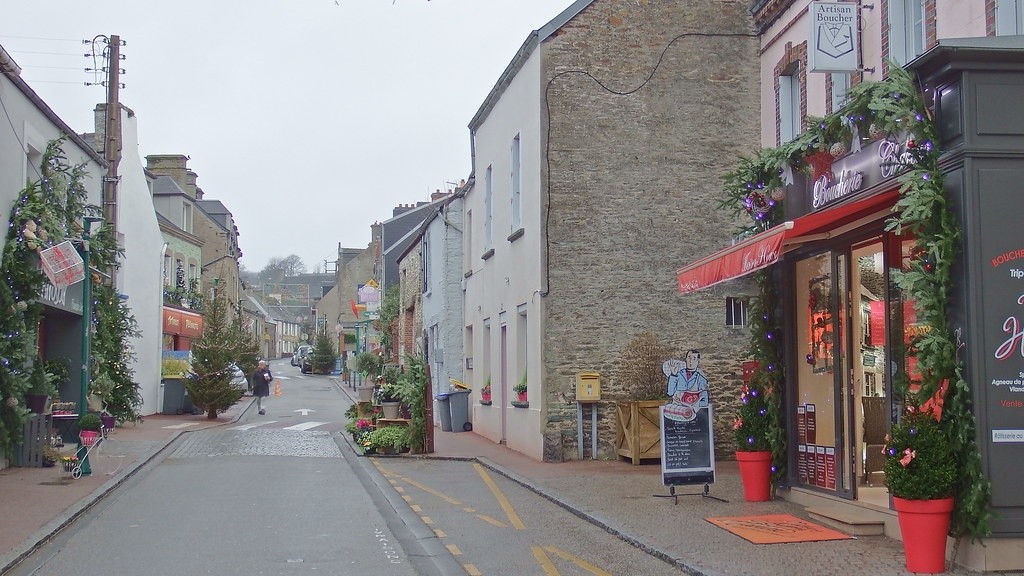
[[880, 389, 953, 573], [341, 353, 424, 454], [614, 331, 667, 466], [481, 376, 491, 400], [513, 373, 527, 401], [77, 415, 101, 444], [31, 445, 62, 466], [93, 373, 118, 429], [733, 382, 774, 501], [52, 401, 77, 415], [25, 365, 48, 413]]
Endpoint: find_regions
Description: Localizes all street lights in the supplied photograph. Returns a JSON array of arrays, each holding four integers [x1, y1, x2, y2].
[[80, 215, 107, 417], [239, 299, 247, 332]]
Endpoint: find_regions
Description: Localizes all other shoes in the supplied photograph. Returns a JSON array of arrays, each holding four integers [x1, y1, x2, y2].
[[259, 411, 264, 415]]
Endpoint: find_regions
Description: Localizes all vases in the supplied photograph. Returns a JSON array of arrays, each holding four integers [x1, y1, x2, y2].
[[63, 463, 79, 472]]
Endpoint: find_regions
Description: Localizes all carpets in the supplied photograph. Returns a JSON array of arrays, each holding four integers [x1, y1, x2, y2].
[[703, 513, 854, 545]]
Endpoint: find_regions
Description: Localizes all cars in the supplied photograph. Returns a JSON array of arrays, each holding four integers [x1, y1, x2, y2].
[[216, 361, 248, 399], [298, 348, 330, 373], [291, 346, 314, 367]]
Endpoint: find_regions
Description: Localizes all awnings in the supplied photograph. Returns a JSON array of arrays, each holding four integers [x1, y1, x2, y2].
[[676, 184, 922, 296]]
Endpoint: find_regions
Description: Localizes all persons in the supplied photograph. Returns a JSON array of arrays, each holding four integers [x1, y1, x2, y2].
[[251, 359, 273, 415]]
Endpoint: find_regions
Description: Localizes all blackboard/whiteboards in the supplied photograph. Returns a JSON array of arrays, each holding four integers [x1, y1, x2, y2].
[[659, 404, 716, 472]]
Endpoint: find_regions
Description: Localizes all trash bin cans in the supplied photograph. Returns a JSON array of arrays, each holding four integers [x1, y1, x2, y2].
[[447, 388, 472, 433], [163, 377, 186, 415], [436, 392, 451, 432], [183, 375, 205, 416]]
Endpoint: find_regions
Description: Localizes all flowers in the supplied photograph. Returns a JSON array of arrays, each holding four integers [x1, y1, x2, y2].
[[357, 418, 372, 435], [60, 456, 81, 464]]
